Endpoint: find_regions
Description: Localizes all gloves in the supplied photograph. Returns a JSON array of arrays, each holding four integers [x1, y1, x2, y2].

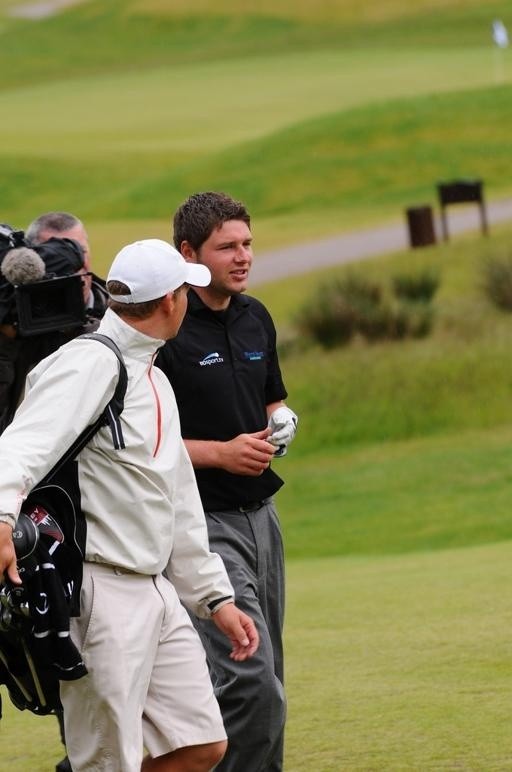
[[266, 407, 298, 458]]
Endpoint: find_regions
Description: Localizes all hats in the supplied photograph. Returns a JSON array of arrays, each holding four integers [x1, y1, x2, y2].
[[106, 238, 212, 305]]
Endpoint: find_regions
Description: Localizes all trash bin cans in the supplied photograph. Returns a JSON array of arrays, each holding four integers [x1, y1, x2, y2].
[[408, 205, 437, 247]]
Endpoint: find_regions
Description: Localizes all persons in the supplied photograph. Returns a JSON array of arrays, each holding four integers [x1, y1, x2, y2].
[[0, 239, 260, 772], [154, 192, 299, 772], [0, 212, 111, 429]]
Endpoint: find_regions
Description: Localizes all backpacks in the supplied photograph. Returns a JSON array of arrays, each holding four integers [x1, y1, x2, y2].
[[0, 461, 88, 716]]
